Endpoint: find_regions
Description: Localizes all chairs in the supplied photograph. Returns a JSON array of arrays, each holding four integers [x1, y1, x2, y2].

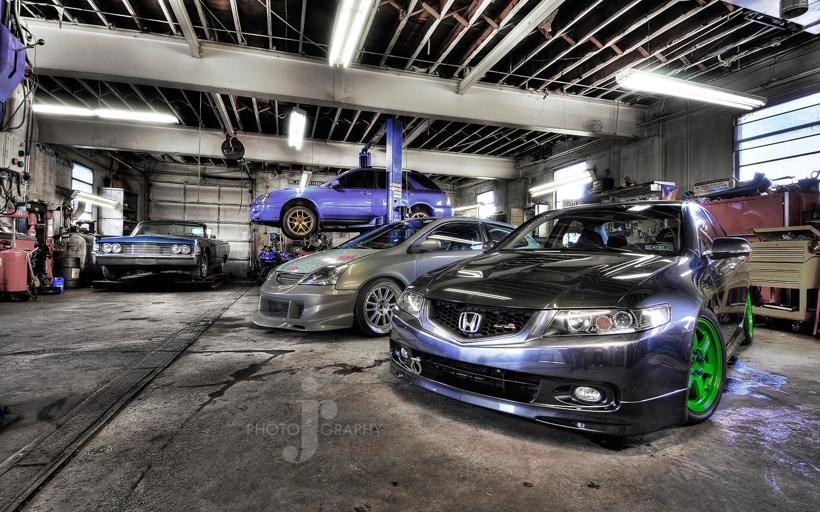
[[606, 235, 628, 247], [653, 228, 678, 246], [577, 232, 604, 248]]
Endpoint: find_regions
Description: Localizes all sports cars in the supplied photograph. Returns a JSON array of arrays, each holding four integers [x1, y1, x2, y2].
[[253, 216, 540, 336], [391, 200, 753, 438]]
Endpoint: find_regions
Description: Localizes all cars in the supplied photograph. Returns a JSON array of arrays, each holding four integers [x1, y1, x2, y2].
[[251, 163, 455, 239]]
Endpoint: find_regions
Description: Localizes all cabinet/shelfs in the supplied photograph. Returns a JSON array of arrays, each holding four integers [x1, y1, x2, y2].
[[522, 204, 548, 238], [99, 187, 138, 236], [700, 191, 820, 305]]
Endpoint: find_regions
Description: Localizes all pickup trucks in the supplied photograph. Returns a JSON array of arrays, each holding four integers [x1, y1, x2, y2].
[[94, 217, 229, 277]]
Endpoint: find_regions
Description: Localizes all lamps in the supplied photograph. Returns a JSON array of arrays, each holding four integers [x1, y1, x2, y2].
[[300, 171, 311, 187], [287, 106, 307, 151], [70, 188, 119, 212], [528, 168, 595, 198], [321, 0, 381, 68], [615, 16, 767, 111]]
